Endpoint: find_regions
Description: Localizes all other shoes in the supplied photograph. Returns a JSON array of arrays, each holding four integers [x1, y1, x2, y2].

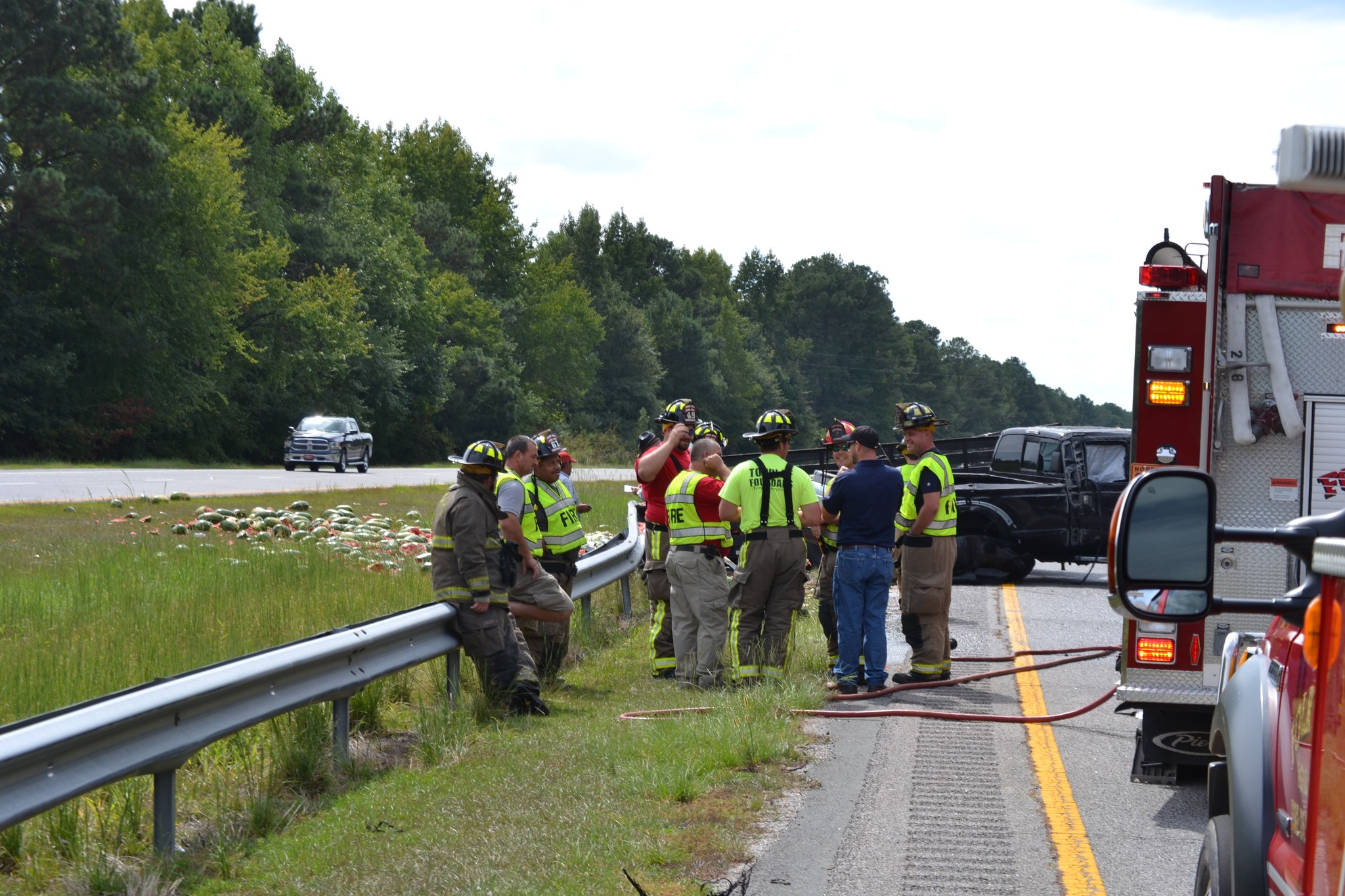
[[651, 667, 676, 679], [698, 684, 725, 693], [892, 671, 951, 684], [831, 683, 858, 695], [677, 677, 695, 690], [826, 679, 837, 689], [731, 677, 780, 690], [867, 684, 893, 697], [856, 675, 865, 686]]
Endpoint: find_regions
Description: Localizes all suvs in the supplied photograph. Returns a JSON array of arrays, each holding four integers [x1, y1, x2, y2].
[[949, 425, 1130, 583], [1100, 462, 1345, 896]]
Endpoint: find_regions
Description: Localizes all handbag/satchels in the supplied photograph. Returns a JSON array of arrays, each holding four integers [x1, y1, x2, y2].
[[501, 539, 522, 588]]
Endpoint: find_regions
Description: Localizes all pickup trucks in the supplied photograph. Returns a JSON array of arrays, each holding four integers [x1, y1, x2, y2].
[[282, 414, 374, 474]]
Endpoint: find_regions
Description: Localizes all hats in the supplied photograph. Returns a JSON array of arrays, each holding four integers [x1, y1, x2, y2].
[[559, 451, 576, 464], [841, 425, 879, 449], [637, 430, 663, 457]]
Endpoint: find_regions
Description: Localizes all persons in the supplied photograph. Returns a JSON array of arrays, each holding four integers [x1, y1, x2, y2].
[[896, 438, 958, 650], [891, 401, 959, 684], [633, 399, 819, 690], [820, 426, 904, 695], [431, 429, 592, 714], [816, 418, 867, 689]]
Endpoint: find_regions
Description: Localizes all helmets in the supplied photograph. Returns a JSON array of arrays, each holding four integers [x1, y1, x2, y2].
[[449, 440, 509, 475], [742, 410, 800, 441], [532, 429, 567, 458], [895, 438, 907, 457], [654, 399, 703, 424], [822, 421, 856, 444], [893, 402, 950, 430], [692, 421, 728, 450]]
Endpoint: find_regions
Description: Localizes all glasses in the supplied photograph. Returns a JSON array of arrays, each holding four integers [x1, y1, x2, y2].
[[831, 445, 849, 452]]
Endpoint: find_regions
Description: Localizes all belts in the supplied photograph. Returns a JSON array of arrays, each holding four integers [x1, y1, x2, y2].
[[645, 522, 668, 532], [746, 529, 804, 541], [843, 544, 883, 549], [670, 546, 720, 557]]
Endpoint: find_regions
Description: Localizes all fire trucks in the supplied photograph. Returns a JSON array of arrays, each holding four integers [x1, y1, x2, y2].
[[1115, 123, 1345, 784]]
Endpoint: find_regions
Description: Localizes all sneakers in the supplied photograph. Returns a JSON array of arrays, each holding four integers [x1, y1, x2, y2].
[[512, 683, 550, 717], [913, 638, 957, 651]]
